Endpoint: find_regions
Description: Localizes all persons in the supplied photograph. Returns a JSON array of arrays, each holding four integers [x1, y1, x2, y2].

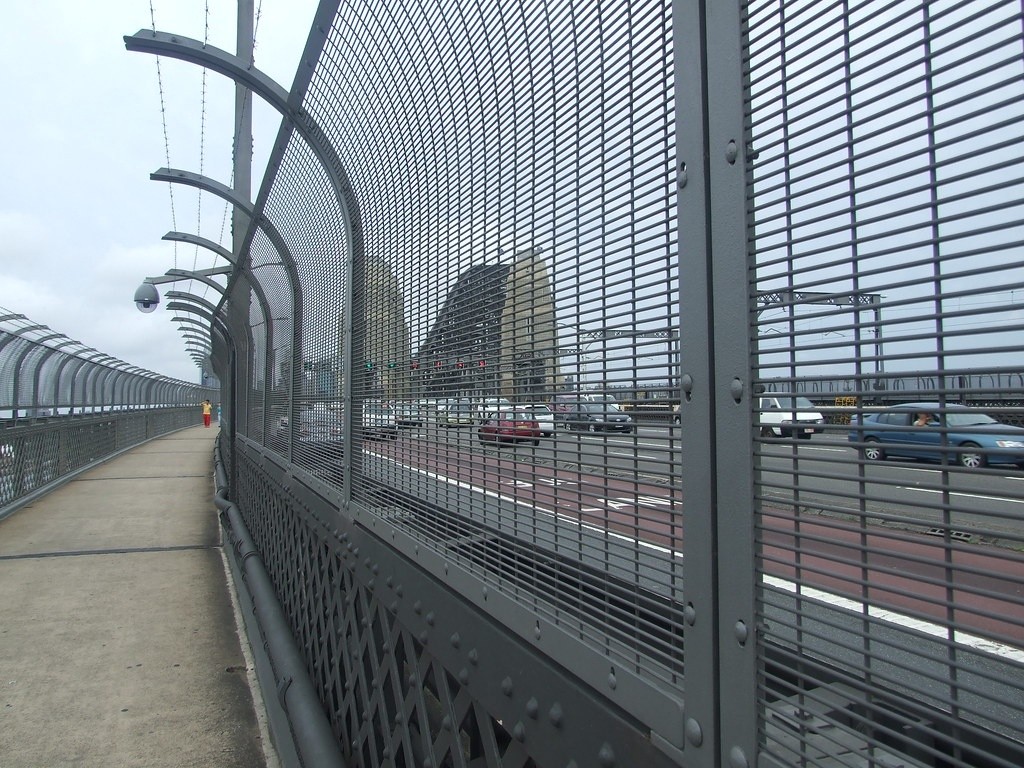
[[203, 400, 212, 427], [914, 414, 935, 426], [216, 402, 221, 427]]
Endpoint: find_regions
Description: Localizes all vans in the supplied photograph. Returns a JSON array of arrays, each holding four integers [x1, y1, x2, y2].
[[760, 392, 824, 439]]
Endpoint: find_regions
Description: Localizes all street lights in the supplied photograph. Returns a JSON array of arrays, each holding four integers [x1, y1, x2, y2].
[[134, 264, 255, 438]]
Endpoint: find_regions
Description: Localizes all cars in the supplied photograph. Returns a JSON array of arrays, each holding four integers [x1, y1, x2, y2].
[[674, 405, 681, 425], [547, 396, 587, 417], [478, 408, 541, 446], [848, 403, 1024, 468], [478, 398, 511, 425], [584, 394, 620, 411], [509, 405, 555, 438], [563, 402, 633, 434], [253, 399, 476, 442]]
